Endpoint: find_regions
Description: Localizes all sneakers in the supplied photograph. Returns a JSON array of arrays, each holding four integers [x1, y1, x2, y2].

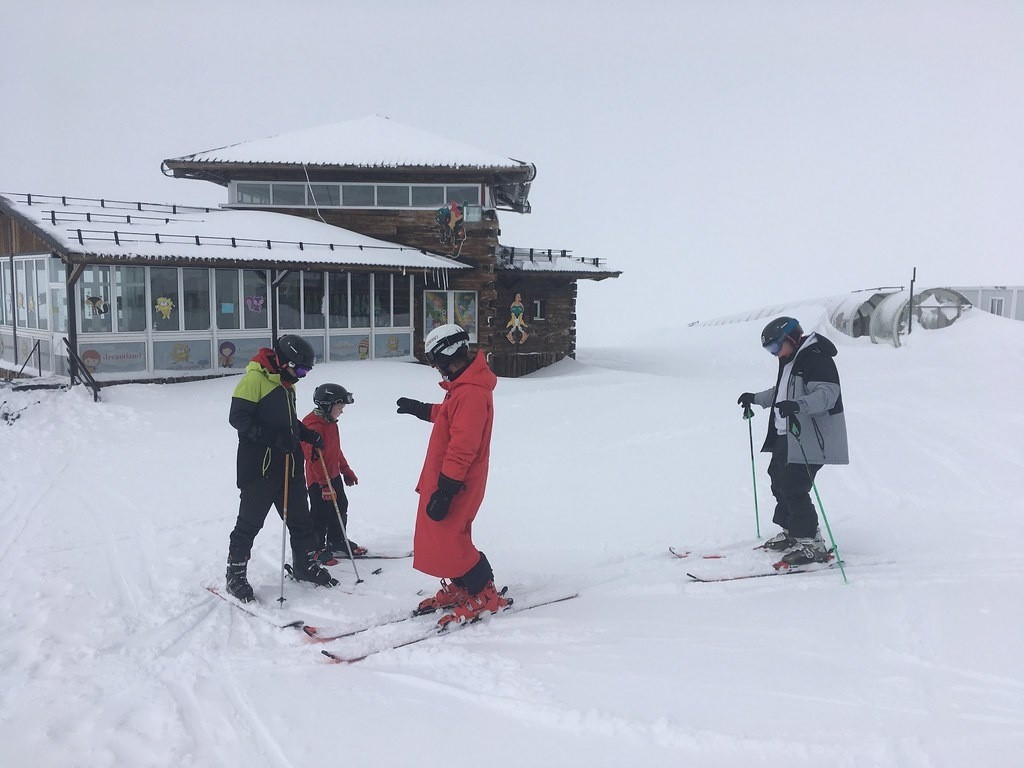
[[782, 543, 828, 563], [454, 584, 496, 616], [329, 541, 368, 554], [314, 550, 340, 566], [437, 585, 463, 606], [765, 536, 799, 551]]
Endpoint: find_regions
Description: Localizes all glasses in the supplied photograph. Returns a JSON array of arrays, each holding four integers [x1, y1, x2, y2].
[[294, 364, 312, 377], [342, 392, 354, 404], [424, 352, 436, 370], [762, 338, 783, 355]]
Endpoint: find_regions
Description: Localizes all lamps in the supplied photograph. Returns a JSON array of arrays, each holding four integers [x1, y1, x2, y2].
[[464, 204, 483, 222]]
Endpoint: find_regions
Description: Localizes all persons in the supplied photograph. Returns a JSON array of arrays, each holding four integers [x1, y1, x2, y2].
[[737, 316, 850, 565], [226, 334, 332, 599], [301, 383, 366, 564], [397, 323, 501, 621]]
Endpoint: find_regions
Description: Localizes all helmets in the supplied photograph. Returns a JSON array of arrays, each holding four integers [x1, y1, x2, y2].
[[274, 334, 314, 367], [313, 383, 348, 405], [425, 324, 470, 357], [762, 317, 803, 346]]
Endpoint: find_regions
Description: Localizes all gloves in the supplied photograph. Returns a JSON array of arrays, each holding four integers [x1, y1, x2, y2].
[[775, 400, 800, 418], [425, 490, 451, 521], [273, 432, 299, 454], [310, 432, 323, 448], [395, 397, 432, 424], [736, 392, 755, 407]]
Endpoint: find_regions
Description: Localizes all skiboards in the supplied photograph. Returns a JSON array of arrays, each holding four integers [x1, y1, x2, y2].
[[670, 539, 845, 583], [198, 563, 424, 628], [303, 585, 580, 664], [327, 547, 415, 574]]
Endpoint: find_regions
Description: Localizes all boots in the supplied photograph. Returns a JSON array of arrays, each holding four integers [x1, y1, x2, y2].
[[225, 554, 253, 598], [289, 538, 331, 585]]
